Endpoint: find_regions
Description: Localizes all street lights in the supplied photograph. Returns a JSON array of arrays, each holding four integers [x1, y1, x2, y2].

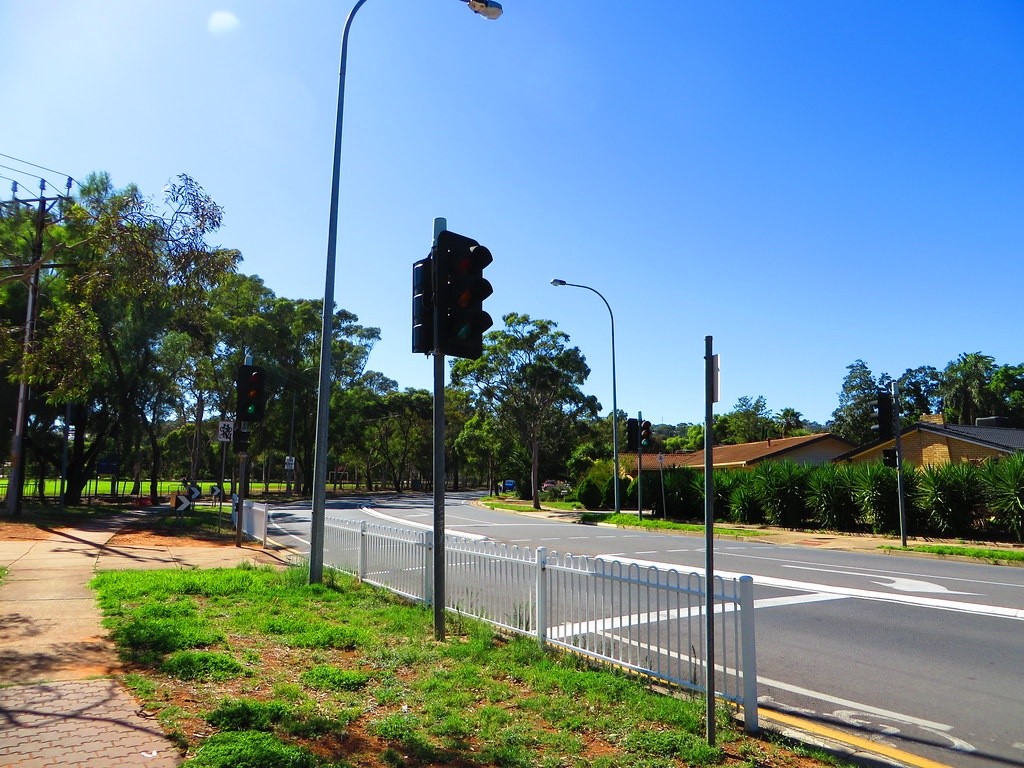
[[550, 278, 621, 516], [308, 0, 505, 588]]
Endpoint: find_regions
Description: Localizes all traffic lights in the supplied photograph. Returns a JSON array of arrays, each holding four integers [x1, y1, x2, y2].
[[638, 420, 651, 449], [437, 231, 494, 360], [883, 447, 897, 469], [868, 393, 893, 442], [236, 364, 266, 422]]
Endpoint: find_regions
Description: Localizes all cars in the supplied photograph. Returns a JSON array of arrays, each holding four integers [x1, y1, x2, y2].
[[505, 480, 515, 491], [544, 479, 555, 490]]
